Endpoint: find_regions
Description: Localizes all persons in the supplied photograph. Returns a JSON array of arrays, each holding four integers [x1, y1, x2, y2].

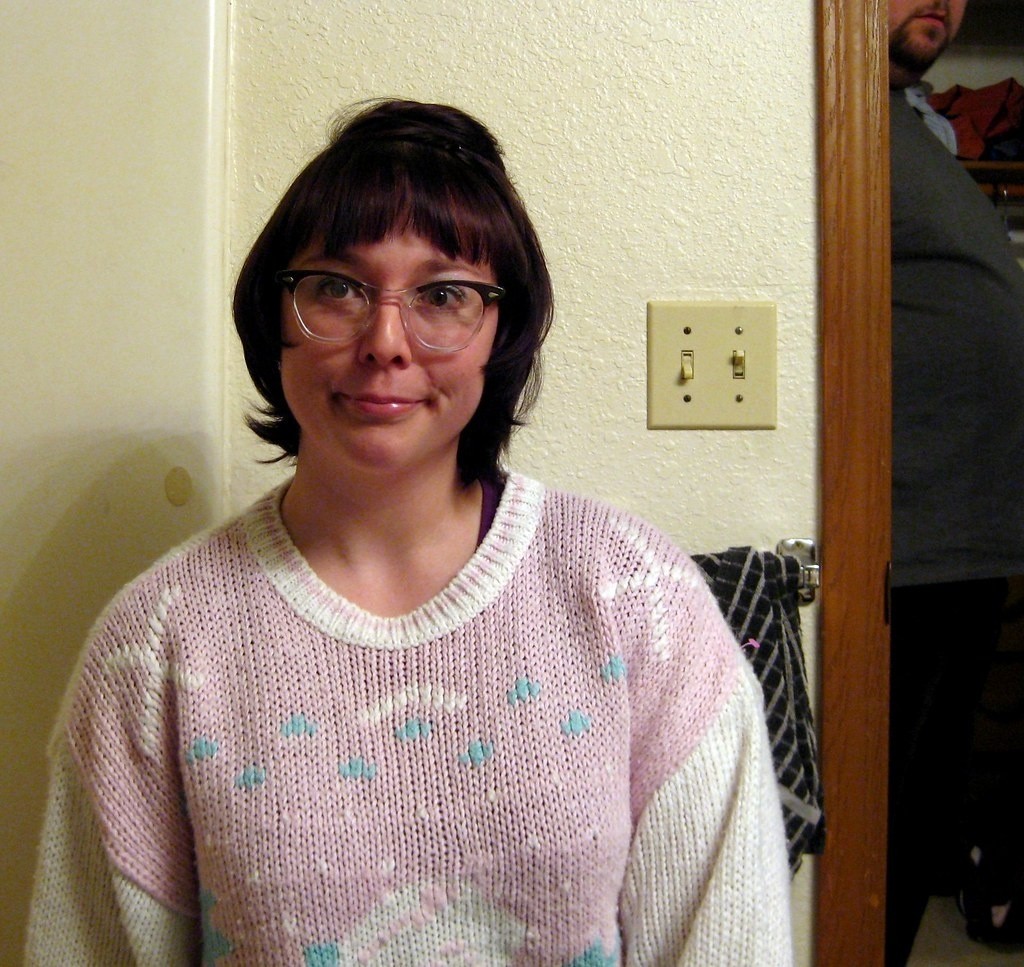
[[21, 101, 792, 966], [887, 1, 1024, 967]]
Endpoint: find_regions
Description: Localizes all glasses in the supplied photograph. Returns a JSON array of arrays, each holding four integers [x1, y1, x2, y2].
[[275, 269, 506, 354]]
